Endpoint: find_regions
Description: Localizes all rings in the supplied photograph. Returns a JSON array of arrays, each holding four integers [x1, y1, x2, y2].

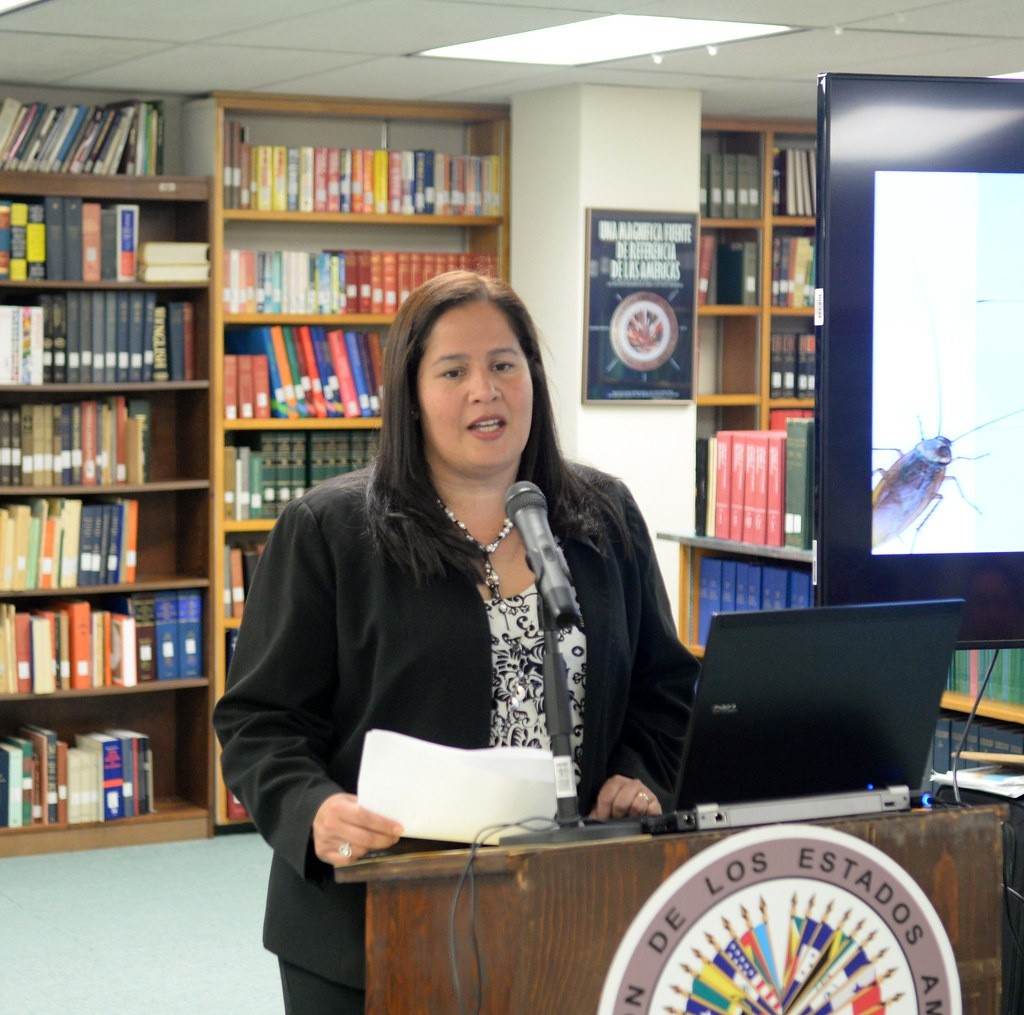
[[639, 793, 648, 801], [338, 842, 352, 856]]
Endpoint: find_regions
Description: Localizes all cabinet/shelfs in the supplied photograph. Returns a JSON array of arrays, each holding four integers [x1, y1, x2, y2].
[[658, 528, 1024, 729], [695, 111, 819, 434], [0, 88, 513, 860]]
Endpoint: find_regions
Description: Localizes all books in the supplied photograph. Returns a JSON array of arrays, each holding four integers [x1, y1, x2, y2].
[[0, 99, 165, 175], [225, 248, 499, 314], [0, 396, 153, 487], [0, 290, 196, 384], [700, 147, 816, 428], [0, 496, 139, 591], [0, 589, 202, 694], [699, 420, 1023, 797], [224, 544, 266, 820], [224, 324, 385, 418], [222, 119, 504, 214], [225, 430, 381, 519], [0, 195, 211, 283], [0, 725, 154, 828]]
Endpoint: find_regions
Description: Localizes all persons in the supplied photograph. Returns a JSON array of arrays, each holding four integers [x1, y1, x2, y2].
[[213, 271, 701, 1015]]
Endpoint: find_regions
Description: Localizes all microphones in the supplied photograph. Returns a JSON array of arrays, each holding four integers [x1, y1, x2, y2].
[[504, 481, 580, 628]]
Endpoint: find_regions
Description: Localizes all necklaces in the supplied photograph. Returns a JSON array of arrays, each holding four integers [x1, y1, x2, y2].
[[436, 502, 513, 600]]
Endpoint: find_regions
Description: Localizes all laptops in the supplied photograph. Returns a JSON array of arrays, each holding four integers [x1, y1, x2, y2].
[[494, 598, 969, 845]]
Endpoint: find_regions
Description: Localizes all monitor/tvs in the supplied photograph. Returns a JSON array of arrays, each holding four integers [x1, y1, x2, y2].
[[813, 73, 1024, 648]]
